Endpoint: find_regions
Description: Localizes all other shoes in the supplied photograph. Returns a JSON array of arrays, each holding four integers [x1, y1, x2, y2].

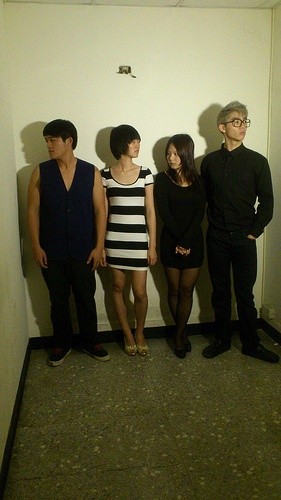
[[46, 342, 71, 366], [76, 341, 110, 361], [241, 343, 280, 363], [202, 345, 231, 358], [184, 339, 191, 352], [175, 348, 186, 358]]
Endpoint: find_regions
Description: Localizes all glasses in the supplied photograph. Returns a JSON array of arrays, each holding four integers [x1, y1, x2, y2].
[[221, 119, 251, 128]]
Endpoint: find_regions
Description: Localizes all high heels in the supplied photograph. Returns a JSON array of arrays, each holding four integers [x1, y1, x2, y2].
[[134, 335, 148, 355], [124, 336, 137, 355]]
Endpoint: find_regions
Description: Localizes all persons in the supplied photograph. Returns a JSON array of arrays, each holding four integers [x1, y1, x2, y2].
[[154, 135, 207, 359], [100, 125, 158, 358], [200, 104, 279, 363], [27, 120, 107, 366]]
